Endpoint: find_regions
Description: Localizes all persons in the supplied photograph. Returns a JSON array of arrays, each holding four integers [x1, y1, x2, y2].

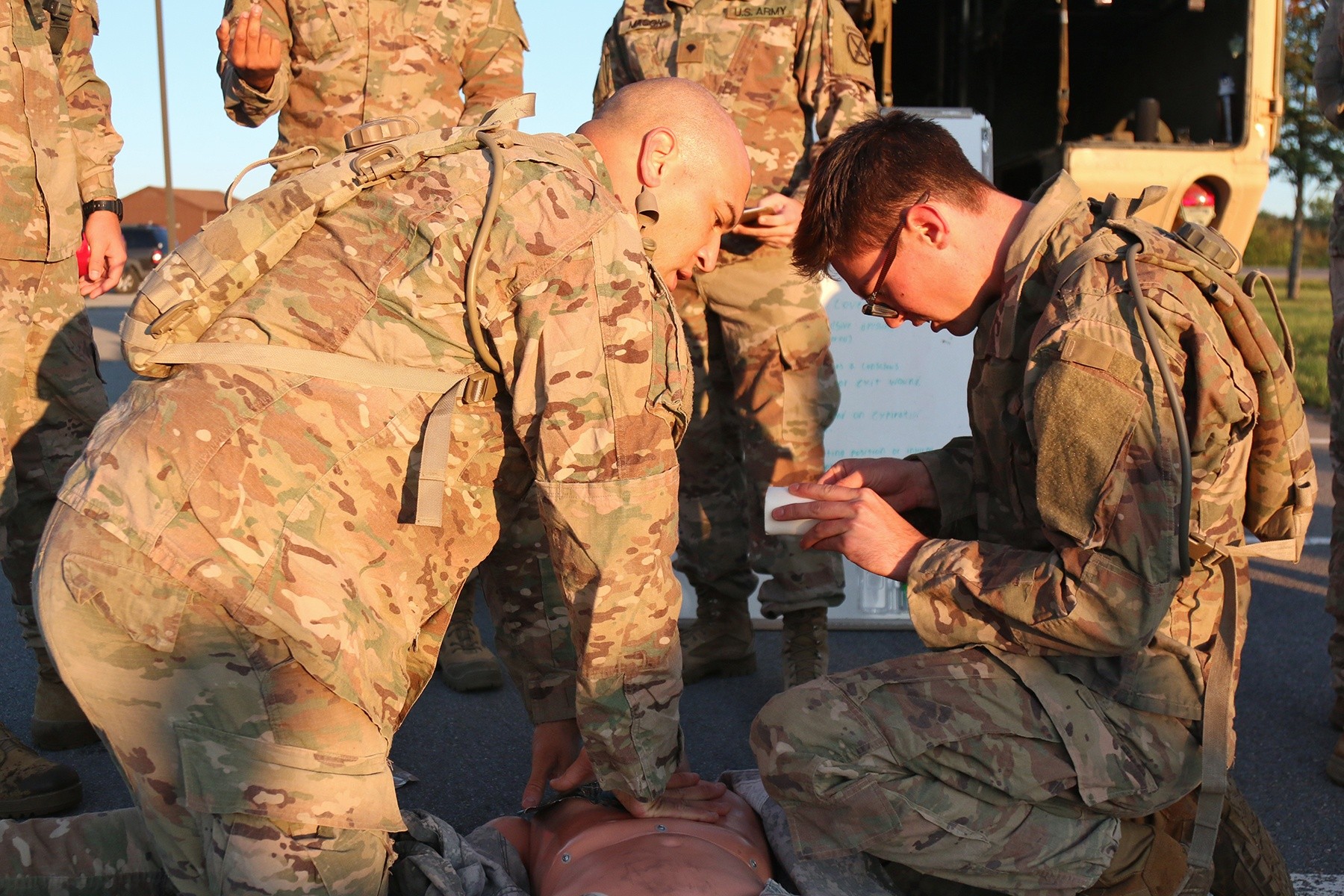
[[214, 1, 576, 696], [1319, 0, 1344, 789], [588, 1, 882, 683], [0, 0, 125, 815], [754, 111, 1317, 895], [387, 744, 890, 896], [1, 75, 756, 896]]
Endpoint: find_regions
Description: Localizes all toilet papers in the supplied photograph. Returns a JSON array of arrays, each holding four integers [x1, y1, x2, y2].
[[760, 486, 820, 536]]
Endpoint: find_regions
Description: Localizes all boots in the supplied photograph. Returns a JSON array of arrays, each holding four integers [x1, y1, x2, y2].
[[438, 574, 505, 692], [780, 604, 830, 689], [0, 720, 85, 818], [678, 588, 759, 686], [1078, 772, 1295, 896], [30, 648, 101, 751]]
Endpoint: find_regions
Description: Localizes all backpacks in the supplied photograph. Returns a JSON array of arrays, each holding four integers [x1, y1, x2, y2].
[[1041, 182, 1318, 868], [118, 90, 605, 528]]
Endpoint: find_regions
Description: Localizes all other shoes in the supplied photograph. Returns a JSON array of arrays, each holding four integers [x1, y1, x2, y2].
[[1325, 690, 1344, 782]]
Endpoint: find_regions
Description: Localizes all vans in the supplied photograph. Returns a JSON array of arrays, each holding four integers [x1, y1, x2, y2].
[[111, 221, 170, 295]]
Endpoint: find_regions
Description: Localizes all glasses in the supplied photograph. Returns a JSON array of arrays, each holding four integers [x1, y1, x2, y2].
[[861, 190, 932, 318]]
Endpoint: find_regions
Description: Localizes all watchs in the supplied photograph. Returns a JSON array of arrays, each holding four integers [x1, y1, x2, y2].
[[79, 198, 123, 227]]
